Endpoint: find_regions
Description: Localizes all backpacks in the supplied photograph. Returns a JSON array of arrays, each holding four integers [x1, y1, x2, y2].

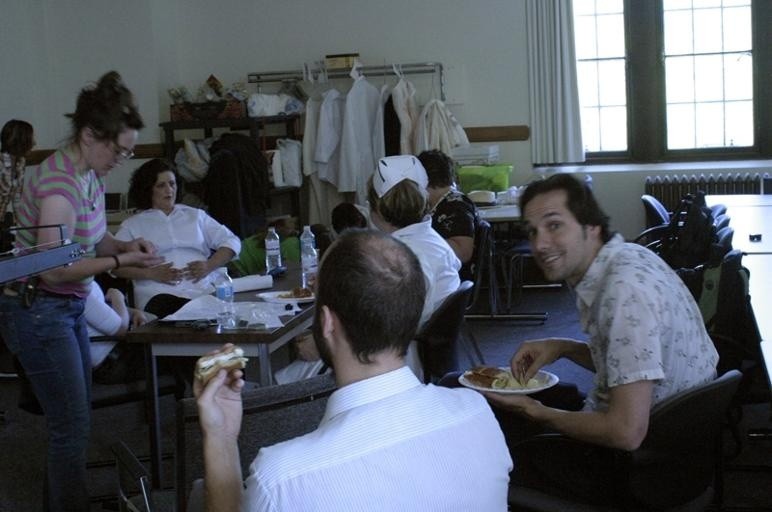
[[658, 189, 714, 269]]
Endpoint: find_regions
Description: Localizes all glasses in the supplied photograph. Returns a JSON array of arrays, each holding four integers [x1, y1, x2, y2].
[[89, 125, 134, 160]]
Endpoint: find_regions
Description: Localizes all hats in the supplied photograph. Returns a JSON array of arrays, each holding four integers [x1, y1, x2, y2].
[[465, 190, 503, 208], [371, 152, 431, 199]]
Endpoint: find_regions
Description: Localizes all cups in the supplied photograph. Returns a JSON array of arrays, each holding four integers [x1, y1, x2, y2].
[[495, 184, 530, 204]]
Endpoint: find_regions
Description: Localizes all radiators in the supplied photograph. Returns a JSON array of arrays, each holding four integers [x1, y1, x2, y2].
[[645, 173, 771, 246]]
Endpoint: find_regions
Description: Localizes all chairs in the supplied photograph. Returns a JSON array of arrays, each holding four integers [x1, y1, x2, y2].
[[14, 193, 772, 511]]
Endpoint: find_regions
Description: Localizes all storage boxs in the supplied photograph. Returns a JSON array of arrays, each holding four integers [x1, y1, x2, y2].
[[456, 162, 514, 195]]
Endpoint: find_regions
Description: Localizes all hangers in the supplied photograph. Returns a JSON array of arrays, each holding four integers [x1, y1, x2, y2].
[[428, 64, 439, 101], [384, 64, 386, 85]]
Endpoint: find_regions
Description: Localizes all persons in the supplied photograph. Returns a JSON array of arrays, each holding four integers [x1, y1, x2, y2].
[[0, 119, 37, 256], [327, 201, 379, 242], [417, 148, 481, 284], [82, 279, 150, 386], [1, 69, 167, 511], [227, 224, 332, 278], [438, 171, 720, 512], [366, 154, 463, 343], [191, 225, 515, 511], [114, 157, 242, 320]]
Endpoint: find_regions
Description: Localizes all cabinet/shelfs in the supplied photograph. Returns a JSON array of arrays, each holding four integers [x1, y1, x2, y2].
[[158, 113, 309, 232]]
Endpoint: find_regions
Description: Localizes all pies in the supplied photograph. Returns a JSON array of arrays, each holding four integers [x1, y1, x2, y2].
[[462, 362, 512, 389], [189, 345, 247, 386]]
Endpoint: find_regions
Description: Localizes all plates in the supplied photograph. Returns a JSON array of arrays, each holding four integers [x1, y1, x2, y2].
[[256, 290, 316, 305], [457, 365, 560, 395]]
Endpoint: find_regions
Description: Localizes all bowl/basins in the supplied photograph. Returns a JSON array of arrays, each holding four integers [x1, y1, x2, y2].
[[184, 101, 227, 121], [468, 191, 494, 206]]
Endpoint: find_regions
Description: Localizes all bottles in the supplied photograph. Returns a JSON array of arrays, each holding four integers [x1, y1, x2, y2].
[[263, 226, 283, 272], [301, 225, 320, 286], [215, 265, 234, 325]]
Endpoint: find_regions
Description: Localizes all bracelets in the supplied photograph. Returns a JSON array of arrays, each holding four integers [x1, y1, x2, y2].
[[112, 255, 120, 269]]
